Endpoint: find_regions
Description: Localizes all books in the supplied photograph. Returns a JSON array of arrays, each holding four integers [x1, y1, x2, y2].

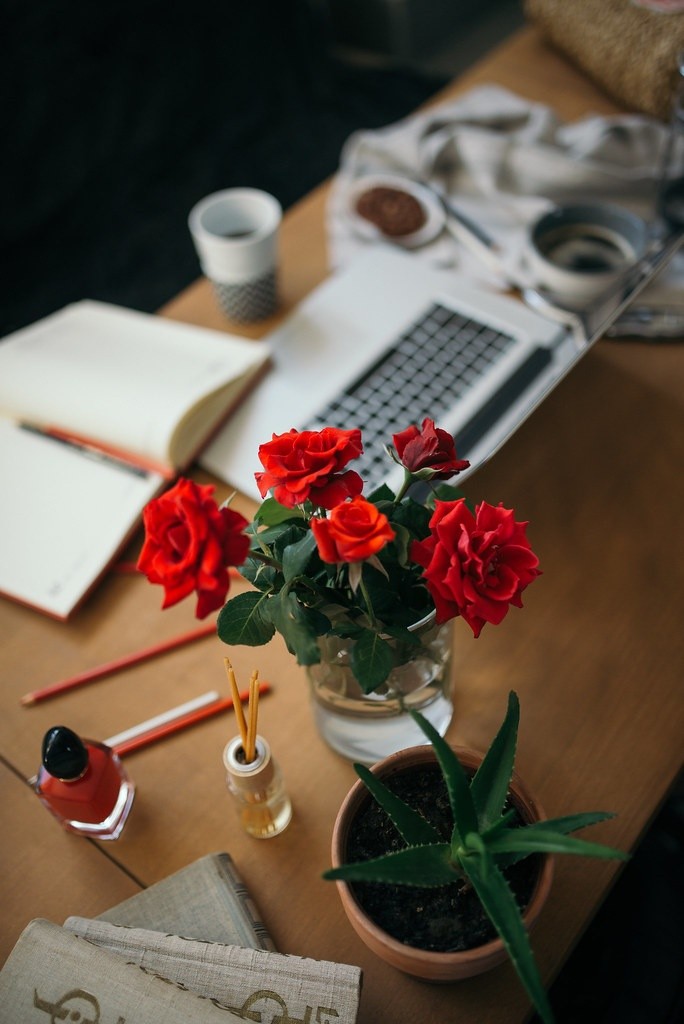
[[61, 915, 363, 1024], [91, 850, 277, 949], [0, 294, 273, 623], [0, 917, 265, 1024]]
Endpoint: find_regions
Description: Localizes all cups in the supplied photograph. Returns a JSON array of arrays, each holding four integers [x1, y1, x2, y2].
[[524, 199, 649, 312], [187, 186, 284, 325]]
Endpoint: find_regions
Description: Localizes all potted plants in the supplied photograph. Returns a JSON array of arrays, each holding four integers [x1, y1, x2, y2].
[[323, 690, 629, 1024]]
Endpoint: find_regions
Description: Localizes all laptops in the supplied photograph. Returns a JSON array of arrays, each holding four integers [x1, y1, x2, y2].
[[190, 217, 684, 536]]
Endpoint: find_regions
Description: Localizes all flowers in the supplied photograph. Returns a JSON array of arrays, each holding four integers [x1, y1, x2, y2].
[[137, 416, 543, 695]]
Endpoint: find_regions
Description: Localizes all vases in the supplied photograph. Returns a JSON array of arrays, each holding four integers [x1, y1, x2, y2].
[[310, 609, 454, 764]]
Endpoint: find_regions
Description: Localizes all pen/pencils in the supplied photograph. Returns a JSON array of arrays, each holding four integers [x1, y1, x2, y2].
[[9, 413, 176, 480], [22, 620, 273, 793]]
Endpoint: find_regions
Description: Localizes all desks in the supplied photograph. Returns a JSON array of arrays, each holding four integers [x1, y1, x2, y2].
[[0, 0, 684, 1024]]
